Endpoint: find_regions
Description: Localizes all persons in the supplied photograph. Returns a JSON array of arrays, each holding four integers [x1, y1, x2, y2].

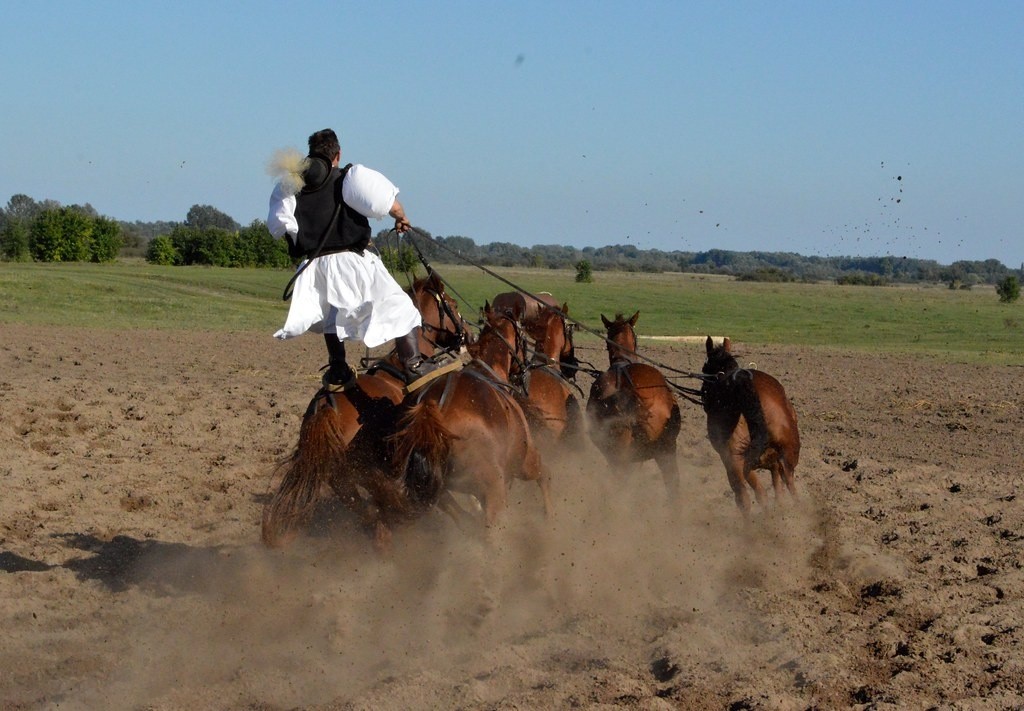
[[266, 128, 443, 394]]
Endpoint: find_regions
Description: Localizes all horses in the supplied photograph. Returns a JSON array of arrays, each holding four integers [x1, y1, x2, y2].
[[584, 310, 681, 505], [380, 299, 557, 546], [701, 335, 801, 520], [510, 301, 586, 518], [259, 269, 479, 559]]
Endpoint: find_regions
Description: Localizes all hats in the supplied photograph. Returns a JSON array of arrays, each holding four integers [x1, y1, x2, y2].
[[293, 151, 333, 193]]
